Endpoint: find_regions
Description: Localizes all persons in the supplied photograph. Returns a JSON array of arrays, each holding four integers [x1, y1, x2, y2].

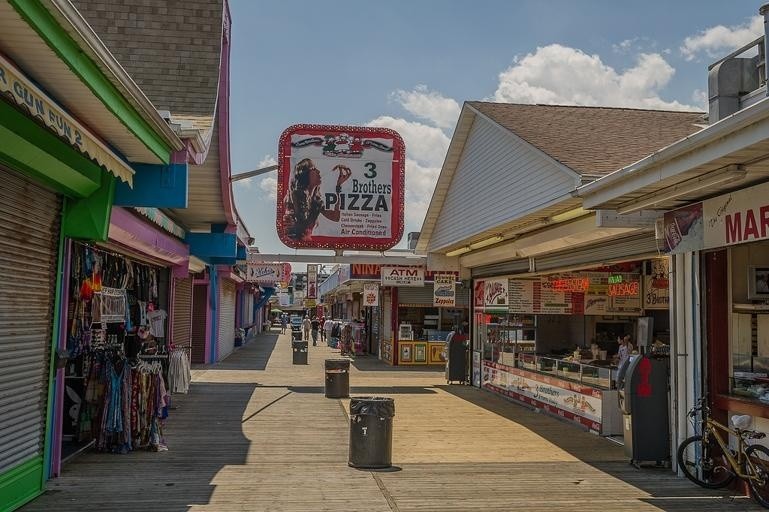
[[311, 315, 341, 346], [280, 313, 288, 335], [282, 157, 352, 241], [302, 314, 311, 341], [617, 334, 634, 359], [446, 326, 456, 344]]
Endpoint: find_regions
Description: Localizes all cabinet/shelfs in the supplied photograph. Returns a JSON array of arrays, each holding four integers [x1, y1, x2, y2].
[[486, 314, 537, 364]]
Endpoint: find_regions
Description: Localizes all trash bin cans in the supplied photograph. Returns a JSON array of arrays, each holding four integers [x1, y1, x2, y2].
[[324, 359, 350, 399], [349, 397, 395, 469], [292, 323, 308, 365]]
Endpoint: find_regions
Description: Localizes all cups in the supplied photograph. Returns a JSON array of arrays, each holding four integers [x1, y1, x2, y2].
[[598, 350, 607, 360], [573, 351, 581, 360]]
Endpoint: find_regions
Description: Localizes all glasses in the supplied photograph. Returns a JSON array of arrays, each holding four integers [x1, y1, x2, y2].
[[309, 167, 317, 171]]
